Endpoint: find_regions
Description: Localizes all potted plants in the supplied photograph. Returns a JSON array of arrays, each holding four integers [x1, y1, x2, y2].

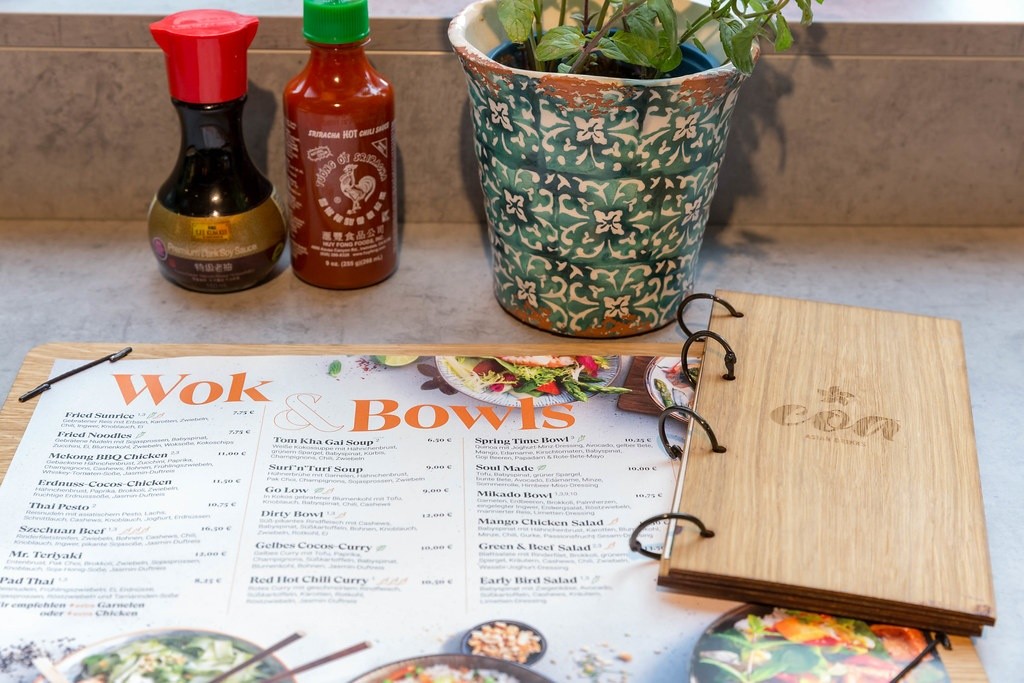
[[445, 1, 819, 340]]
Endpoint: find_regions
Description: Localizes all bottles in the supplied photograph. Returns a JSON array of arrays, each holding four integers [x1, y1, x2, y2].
[[147, 9, 289, 293], [283, 0, 400, 291]]
